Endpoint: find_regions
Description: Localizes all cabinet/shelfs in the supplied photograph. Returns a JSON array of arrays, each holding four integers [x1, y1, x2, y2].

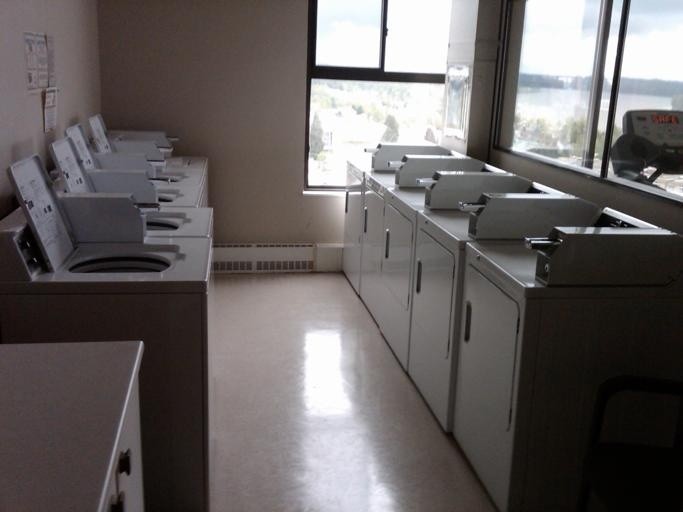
[[101, 372, 144, 511]]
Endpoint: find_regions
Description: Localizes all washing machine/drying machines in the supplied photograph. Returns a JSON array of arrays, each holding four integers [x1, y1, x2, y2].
[[1, 111, 215, 511], [341, 143, 682, 512]]
